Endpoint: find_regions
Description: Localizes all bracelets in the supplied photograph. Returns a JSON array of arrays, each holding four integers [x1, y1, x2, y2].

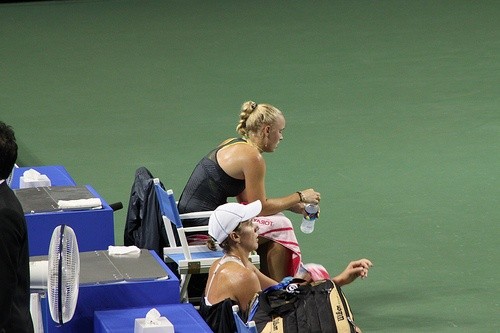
[[297, 191, 303, 201]]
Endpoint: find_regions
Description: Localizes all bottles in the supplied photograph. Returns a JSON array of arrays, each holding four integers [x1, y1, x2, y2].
[[300, 202, 319, 234]]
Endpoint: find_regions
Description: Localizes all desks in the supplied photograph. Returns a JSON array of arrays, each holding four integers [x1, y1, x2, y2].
[[12, 164, 212, 333]]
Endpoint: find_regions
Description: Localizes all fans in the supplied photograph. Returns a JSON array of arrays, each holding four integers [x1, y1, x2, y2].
[[28, 224, 80, 333]]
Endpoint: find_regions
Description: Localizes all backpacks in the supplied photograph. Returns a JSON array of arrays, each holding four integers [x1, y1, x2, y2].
[[248, 277, 362, 333]]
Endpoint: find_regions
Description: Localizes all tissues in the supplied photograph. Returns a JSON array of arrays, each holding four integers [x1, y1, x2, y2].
[[20, 169, 51, 189], [135, 308, 174, 333]]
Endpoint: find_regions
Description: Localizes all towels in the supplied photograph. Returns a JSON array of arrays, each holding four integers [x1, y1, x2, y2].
[[57, 198, 102, 209], [109, 246, 140, 255]]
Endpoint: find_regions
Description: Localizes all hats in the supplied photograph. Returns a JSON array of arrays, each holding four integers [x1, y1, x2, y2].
[[209, 200, 262, 245]]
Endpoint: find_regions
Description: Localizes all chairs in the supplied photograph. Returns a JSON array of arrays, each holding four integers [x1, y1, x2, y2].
[[125, 166, 362, 333]]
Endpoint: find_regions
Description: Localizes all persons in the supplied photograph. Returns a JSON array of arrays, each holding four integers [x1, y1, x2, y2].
[[0, 121, 35, 333], [177, 101, 321, 284], [203, 200, 373, 323]]
